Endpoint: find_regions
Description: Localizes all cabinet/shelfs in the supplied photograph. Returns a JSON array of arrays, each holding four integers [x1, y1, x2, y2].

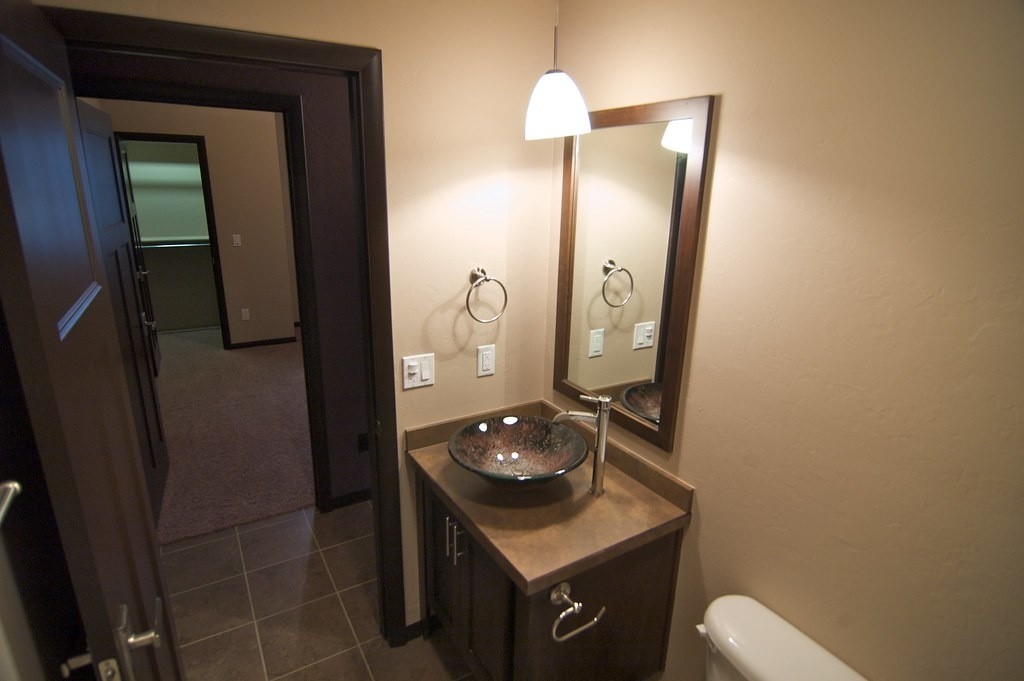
[[416, 482, 682, 681]]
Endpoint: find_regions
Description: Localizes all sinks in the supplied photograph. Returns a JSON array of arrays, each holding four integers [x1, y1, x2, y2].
[[622, 382, 662, 426], [447, 415, 589, 495]]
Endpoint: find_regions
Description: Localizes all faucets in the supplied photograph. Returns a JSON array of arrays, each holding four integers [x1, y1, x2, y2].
[[552, 394, 613, 497]]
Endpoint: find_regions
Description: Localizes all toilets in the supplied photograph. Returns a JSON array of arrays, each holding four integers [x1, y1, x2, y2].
[[694, 593, 866, 681]]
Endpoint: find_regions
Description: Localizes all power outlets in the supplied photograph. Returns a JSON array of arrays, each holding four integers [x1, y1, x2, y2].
[[589, 327, 605, 358], [477, 344, 496, 378]]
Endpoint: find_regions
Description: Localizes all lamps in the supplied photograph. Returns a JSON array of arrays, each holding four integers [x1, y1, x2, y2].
[[524, 0, 593, 140], [660, 119, 693, 157]]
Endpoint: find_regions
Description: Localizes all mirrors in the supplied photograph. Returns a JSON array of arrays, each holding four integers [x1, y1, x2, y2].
[[552, 94, 716, 452]]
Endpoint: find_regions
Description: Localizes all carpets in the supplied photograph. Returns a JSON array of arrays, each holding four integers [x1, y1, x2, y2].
[[155, 345, 317, 547]]
